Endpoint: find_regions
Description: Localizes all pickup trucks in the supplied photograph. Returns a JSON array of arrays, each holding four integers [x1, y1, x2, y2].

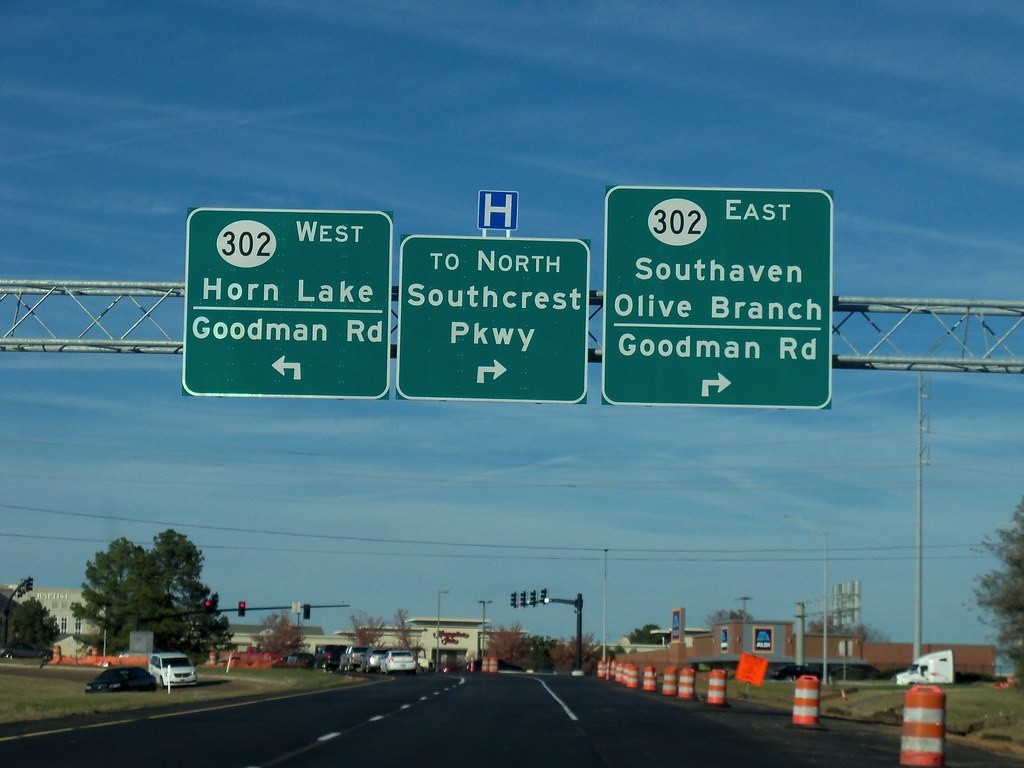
[[219, 646, 283, 669]]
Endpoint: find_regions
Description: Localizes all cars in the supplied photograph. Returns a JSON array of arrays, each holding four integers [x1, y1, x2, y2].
[[380, 649, 418, 675], [0, 640, 54, 662], [442, 661, 470, 674], [288, 653, 316, 669], [768, 649, 908, 682], [360, 647, 393, 674], [85, 666, 158, 694]]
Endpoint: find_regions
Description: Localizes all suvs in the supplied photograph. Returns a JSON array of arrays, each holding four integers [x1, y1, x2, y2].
[[337, 646, 371, 673], [313, 644, 348, 672]]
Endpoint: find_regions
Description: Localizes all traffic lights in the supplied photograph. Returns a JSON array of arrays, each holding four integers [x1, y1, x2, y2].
[[541, 588, 547, 605], [530, 590, 536, 606], [205, 599, 213, 614], [510, 592, 516, 608], [238, 601, 245, 617], [520, 591, 526, 607], [27, 576, 34, 591]]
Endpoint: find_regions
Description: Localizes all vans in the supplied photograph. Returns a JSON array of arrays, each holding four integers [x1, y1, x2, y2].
[[147, 651, 198, 689]]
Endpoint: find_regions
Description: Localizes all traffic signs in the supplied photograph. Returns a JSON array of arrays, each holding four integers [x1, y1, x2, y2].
[[602, 185, 834, 410], [182, 207, 394, 399], [395, 234, 589, 405]]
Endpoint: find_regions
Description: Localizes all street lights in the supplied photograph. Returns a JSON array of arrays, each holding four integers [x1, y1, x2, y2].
[[783, 512, 828, 686], [478, 600, 492, 657], [602, 549, 610, 661], [435, 589, 450, 673]]
